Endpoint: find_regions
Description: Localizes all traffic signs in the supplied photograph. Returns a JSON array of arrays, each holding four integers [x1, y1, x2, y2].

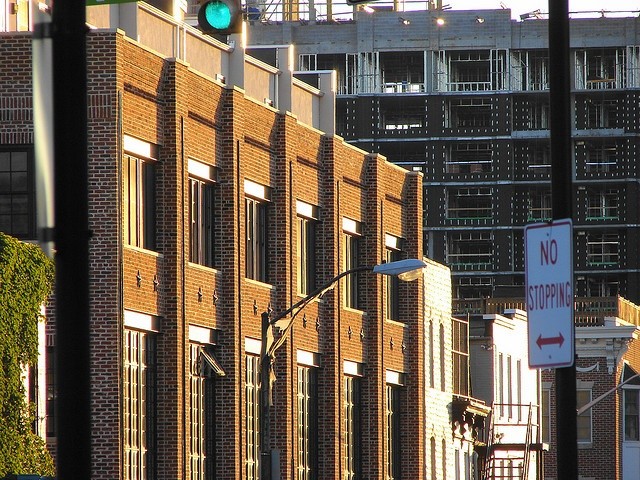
[[524, 219, 577, 369]]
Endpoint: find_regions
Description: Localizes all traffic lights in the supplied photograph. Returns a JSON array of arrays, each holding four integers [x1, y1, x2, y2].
[[198, 0, 242, 35]]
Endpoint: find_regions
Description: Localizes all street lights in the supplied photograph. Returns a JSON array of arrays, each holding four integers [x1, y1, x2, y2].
[[261, 259, 426, 480]]
